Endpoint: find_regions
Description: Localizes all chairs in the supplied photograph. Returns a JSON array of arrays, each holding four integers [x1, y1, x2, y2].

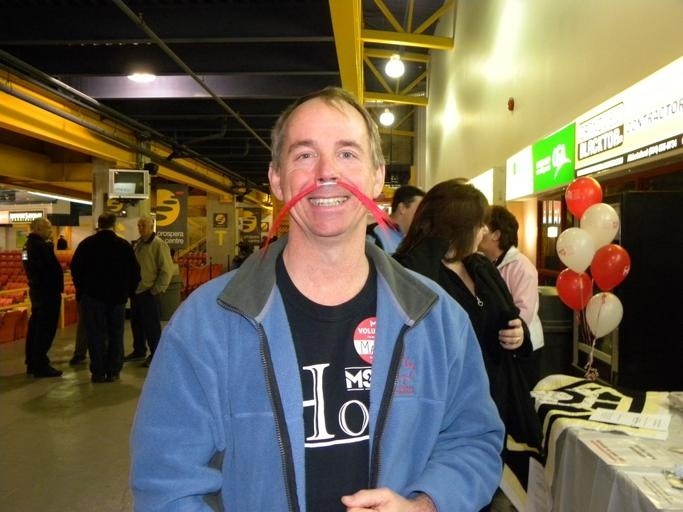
[[0, 250, 223, 341]]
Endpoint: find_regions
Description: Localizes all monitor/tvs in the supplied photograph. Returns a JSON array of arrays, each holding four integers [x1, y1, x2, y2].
[[109, 169, 149, 199]]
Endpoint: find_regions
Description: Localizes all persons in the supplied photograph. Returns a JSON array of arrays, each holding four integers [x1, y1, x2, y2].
[[70, 309, 87, 365], [70, 214, 142, 383], [391, 178, 543, 493], [124, 211, 172, 368], [22, 219, 64, 377], [479, 204, 554, 383], [368, 185, 427, 258], [127, 86, 507, 511]]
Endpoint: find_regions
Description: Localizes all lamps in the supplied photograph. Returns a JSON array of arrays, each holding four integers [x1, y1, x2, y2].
[[385, 52, 404, 78], [380, 106, 394, 125], [127, 61, 156, 84]]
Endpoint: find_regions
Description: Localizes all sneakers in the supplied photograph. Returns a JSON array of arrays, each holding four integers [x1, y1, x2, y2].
[[143, 355, 152, 367], [122, 352, 147, 361]]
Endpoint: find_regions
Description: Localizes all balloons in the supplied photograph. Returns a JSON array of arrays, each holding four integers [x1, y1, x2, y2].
[[557, 227, 596, 275], [557, 267, 594, 310], [586, 291, 625, 339], [590, 244, 633, 292], [566, 175, 602, 219], [581, 203, 619, 249]]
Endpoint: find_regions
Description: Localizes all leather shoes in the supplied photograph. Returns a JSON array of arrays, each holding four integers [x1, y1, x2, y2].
[[107, 365, 122, 375], [93, 369, 111, 382], [33, 362, 63, 377], [27, 363, 36, 373], [70, 355, 86, 365]]
